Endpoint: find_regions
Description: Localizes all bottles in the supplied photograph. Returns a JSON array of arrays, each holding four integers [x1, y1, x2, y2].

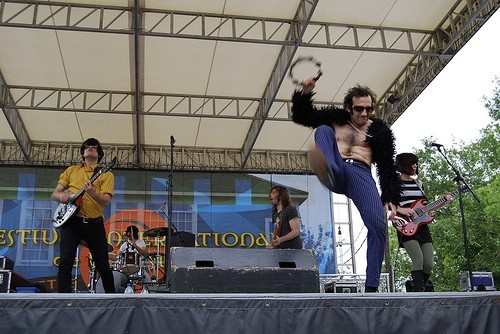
[[141, 281, 149, 294], [124, 283, 134, 294]]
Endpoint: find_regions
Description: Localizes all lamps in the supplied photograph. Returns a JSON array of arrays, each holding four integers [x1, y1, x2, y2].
[[387, 94, 400, 104]]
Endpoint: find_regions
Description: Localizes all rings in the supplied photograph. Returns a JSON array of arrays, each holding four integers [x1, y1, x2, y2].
[[449, 198, 453, 201]]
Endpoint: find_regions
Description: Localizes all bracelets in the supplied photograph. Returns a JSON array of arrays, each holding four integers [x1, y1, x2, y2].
[[136, 246, 139, 250]]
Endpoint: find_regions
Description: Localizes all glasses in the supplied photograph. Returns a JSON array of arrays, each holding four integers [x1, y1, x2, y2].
[[85, 143, 99, 148], [352, 104, 374, 113]]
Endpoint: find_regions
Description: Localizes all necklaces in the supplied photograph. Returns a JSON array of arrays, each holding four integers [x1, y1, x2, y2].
[[348, 121, 373, 137]]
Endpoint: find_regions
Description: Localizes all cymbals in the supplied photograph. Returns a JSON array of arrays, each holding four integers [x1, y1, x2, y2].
[[143, 227, 174, 237], [288, 56, 324, 86], [145, 252, 164, 256], [107, 242, 113, 253]]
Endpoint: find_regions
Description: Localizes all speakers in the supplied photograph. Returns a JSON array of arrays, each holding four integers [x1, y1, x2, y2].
[[169, 247, 321, 294]]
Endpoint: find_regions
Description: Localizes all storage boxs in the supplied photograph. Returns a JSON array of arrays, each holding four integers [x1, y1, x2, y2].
[[0, 255, 8, 270], [0, 270, 13, 293]]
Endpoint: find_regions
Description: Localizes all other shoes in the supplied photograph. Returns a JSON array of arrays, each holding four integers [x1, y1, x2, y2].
[[363, 284, 381, 293], [307, 147, 338, 192]]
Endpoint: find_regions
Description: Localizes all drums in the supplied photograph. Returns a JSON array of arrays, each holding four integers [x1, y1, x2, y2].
[[95, 270, 132, 294], [117, 252, 140, 275], [127, 267, 142, 280]]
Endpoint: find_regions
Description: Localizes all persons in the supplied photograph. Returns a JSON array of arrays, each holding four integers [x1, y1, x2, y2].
[[291, 79, 405, 292], [389, 153, 453, 292], [51, 137, 117, 293], [118, 225, 151, 294], [269, 186, 303, 250]]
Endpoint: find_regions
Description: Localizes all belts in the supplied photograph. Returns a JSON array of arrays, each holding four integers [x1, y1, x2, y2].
[[344, 158, 362, 165]]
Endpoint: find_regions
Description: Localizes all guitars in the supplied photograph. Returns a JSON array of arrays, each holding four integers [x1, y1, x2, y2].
[[52, 156, 115, 228], [272, 222, 277, 249], [391, 185, 470, 236]]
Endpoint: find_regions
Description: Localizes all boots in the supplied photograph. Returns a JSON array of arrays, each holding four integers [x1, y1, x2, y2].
[[423, 272, 431, 291], [410, 270, 423, 292]]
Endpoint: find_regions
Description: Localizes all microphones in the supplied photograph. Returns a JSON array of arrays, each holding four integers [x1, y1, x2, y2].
[[429, 142, 443, 147], [171, 136, 175, 144], [156, 202, 166, 214]]
